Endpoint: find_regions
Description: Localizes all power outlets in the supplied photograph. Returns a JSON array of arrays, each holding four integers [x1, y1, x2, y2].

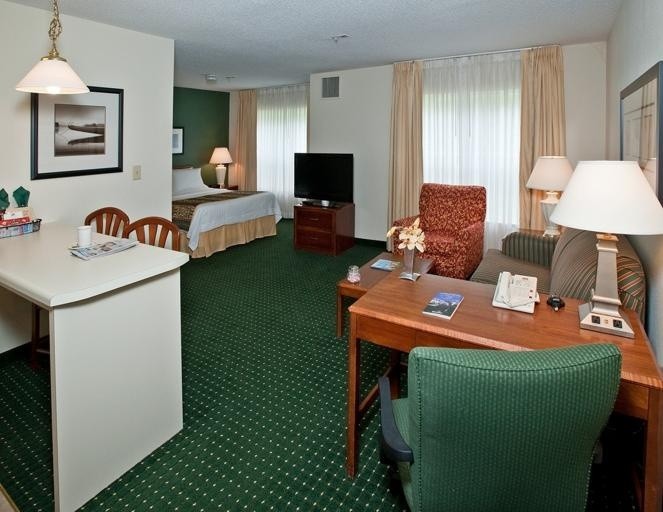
[[132, 165, 141, 180]]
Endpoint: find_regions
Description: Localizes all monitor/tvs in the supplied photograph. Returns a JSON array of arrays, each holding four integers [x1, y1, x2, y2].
[[294, 153, 354, 210]]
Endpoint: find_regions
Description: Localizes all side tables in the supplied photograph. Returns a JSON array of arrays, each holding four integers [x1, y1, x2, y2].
[[519, 228, 562, 238]]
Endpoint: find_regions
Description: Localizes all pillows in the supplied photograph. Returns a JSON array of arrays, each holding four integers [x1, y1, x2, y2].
[[172, 168, 211, 197]]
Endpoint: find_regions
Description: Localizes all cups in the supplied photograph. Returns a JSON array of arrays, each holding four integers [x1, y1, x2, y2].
[[77, 225, 93, 247], [33, 219, 42, 232]]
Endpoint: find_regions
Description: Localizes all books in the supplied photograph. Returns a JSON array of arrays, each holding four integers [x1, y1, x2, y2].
[[422, 293, 464, 320], [371, 259, 401, 271]]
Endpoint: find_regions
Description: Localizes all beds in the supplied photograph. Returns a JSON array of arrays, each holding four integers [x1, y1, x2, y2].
[[172, 165, 282, 259]]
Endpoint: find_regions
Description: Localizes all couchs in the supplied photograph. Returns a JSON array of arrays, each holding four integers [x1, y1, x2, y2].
[[378, 344, 622, 512], [468, 227, 647, 328], [392, 183, 486, 280]]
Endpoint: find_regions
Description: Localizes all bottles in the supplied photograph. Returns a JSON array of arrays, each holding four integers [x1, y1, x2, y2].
[[347, 265, 361, 283]]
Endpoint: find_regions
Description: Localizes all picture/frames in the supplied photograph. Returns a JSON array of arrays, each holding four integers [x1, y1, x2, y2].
[[30, 85, 124, 181], [172, 127, 185, 155]]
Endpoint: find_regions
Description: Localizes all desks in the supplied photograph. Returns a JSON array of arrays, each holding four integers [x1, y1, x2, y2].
[[347, 273, 663, 510]]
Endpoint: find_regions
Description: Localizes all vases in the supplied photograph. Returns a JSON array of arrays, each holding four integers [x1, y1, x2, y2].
[[399, 248, 421, 282]]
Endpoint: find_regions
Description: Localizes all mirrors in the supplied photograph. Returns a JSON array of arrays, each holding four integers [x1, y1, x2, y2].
[[619, 61, 663, 202]]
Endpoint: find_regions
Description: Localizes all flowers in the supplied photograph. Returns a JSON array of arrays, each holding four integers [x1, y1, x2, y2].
[[386, 217, 428, 254]]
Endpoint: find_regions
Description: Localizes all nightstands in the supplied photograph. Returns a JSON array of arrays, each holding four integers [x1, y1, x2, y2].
[[209, 185, 239, 190]]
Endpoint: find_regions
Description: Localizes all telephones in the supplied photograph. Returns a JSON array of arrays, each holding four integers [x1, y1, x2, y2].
[[492, 272, 537, 313]]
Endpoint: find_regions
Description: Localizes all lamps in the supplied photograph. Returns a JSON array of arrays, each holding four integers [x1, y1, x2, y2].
[[525, 155, 574, 238], [14, 0, 90, 94], [549, 160, 663, 338], [209, 147, 233, 189]]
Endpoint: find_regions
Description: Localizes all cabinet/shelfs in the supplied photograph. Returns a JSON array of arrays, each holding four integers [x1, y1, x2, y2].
[[293, 201, 355, 257]]
